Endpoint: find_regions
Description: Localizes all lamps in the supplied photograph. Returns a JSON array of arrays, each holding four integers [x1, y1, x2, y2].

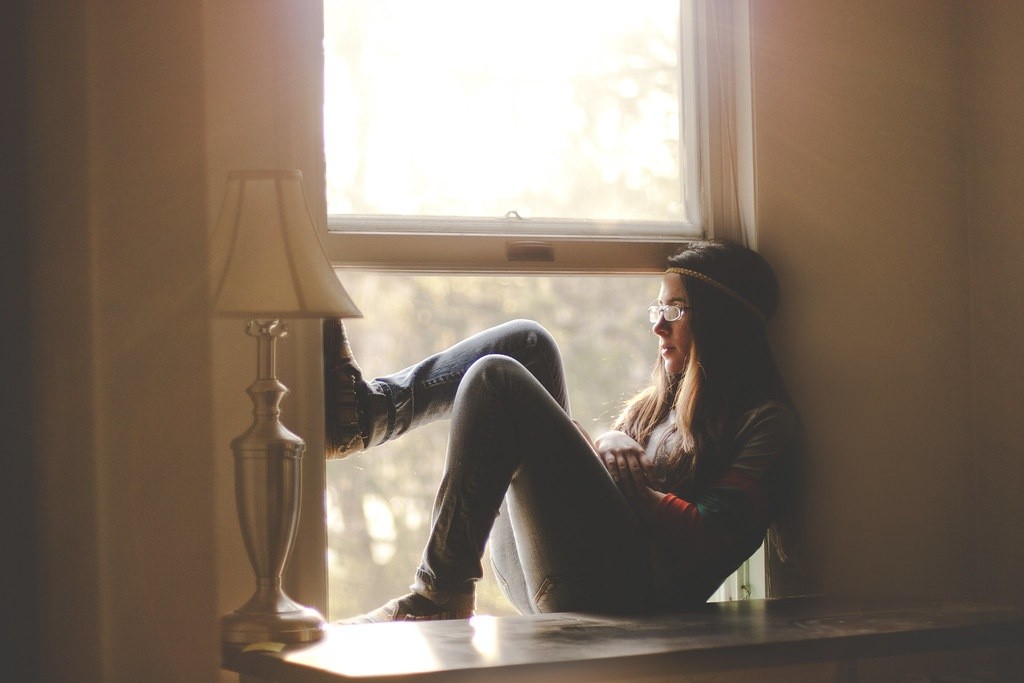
[[206, 169, 364, 643]]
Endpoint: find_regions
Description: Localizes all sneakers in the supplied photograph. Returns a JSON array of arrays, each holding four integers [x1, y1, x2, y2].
[[324, 318, 366, 461], [333, 586, 474, 621]]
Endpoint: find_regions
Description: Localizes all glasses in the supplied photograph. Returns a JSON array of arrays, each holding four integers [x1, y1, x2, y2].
[[649, 303, 695, 323]]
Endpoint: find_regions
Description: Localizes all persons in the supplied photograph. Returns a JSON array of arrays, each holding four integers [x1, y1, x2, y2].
[[325, 242, 794, 623]]
